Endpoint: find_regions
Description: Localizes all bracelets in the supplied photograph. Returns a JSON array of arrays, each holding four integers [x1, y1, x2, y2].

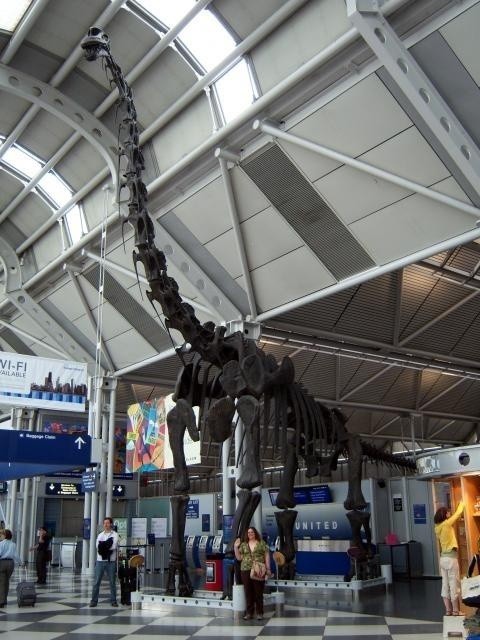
[[266, 569, 272, 571]]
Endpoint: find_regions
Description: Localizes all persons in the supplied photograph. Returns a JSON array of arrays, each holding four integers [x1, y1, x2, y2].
[[233, 525, 272, 620], [0, 528, 23, 608], [89, 517, 119, 607], [463, 532, 480, 616], [0, 520, 7, 542], [433, 496, 467, 616], [30, 526, 49, 585]]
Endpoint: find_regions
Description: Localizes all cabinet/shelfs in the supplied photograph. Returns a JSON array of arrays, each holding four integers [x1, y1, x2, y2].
[[432, 470, 480, 634]]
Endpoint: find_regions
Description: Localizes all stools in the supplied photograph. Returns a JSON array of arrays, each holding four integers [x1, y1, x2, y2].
[[443, 615, 470, 638]]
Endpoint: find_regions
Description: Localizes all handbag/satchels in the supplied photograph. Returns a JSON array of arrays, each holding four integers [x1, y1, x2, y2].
[[248, 561, 267, 581], [460, 574, 480, 609]]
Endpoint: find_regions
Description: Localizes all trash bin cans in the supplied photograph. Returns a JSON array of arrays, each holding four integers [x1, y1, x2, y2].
[[118, 566, 140, 605]]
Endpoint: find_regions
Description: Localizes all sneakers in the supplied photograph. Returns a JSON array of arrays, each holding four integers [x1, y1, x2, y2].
[[242, 613, 264, 620], [89, 602, 119, 607], [35, 580, 46, 584]]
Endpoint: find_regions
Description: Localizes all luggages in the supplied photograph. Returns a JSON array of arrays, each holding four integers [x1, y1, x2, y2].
[[15, 581, 36, 608]]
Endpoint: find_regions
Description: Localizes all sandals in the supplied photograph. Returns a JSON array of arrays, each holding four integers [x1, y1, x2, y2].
[[452, 610, 467, 616], [445, 608, 452, 616]]
